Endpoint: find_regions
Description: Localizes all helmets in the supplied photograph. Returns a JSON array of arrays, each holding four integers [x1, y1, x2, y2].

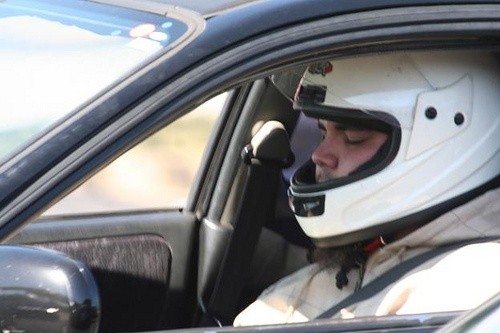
[[285, 50, 500, 248]]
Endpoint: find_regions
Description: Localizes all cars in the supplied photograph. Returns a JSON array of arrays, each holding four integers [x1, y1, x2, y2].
[[1, 0, 499, 333]]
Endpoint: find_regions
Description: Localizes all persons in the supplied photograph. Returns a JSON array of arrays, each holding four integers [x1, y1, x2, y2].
[[233, 47, 499, 327]]
